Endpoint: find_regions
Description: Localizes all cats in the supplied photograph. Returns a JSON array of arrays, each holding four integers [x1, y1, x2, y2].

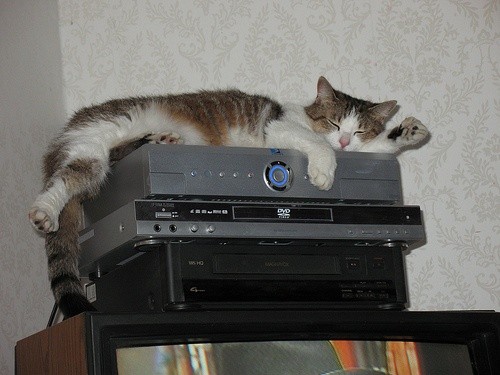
[[29, 76, 431, 319]]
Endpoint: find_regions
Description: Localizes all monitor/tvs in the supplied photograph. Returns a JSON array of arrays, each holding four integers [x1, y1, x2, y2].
[[88, 312, 500, 375]]
